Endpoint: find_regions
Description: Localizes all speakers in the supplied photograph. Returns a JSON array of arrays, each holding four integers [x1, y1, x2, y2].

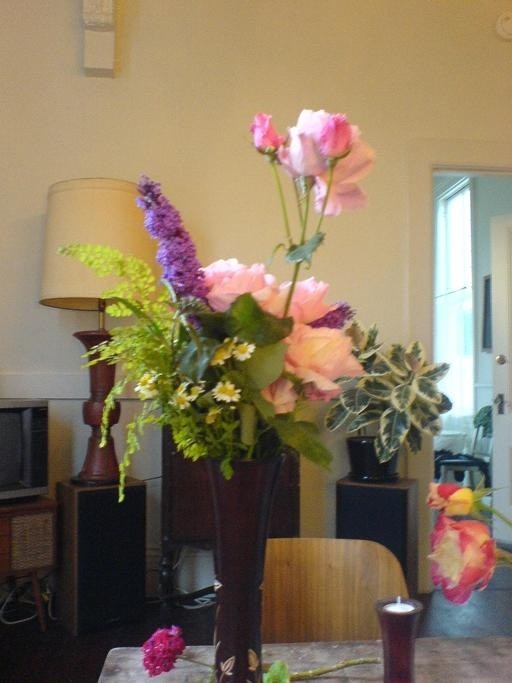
[[335, 475, 419, 595], [55, 476, 147, 639]]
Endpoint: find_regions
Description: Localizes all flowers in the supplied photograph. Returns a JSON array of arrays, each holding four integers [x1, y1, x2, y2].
[[57, 104, 366, 506], [424, 474, 511, 606], [140, 625, 381, 681]]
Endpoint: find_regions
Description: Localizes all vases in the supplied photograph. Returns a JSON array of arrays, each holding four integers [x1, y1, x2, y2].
[[201, 456, 283, 682]]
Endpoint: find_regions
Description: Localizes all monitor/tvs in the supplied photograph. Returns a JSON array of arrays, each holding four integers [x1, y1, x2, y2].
[[0, 397, 50, 500]]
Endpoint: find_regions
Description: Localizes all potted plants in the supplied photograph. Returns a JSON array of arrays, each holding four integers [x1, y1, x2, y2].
[[323, 321, 452, 483]]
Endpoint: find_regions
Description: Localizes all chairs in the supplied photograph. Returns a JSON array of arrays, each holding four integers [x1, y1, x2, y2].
[[438, 406, 492, 487], [259, 536, 410, 641]]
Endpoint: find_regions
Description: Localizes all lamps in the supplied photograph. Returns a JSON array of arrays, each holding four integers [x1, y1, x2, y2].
[[37, 174, 160, 489]]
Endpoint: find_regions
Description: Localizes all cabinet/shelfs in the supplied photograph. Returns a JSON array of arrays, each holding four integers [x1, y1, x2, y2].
[[157, 423, 300, 614], [0, 497, 57, 633]]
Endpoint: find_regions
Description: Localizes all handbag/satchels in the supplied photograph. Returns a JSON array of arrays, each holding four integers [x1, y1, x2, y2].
[[171, 541, 215, 595]]
